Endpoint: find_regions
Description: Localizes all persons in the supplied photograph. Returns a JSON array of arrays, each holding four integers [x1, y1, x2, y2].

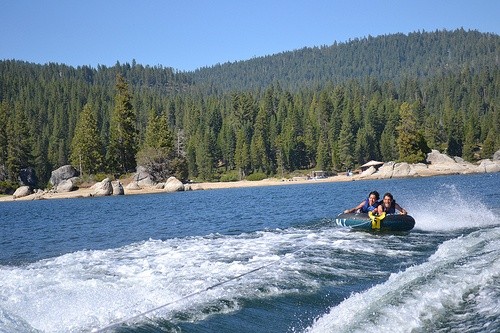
[[377, 192, 407, 215], [344, 190, 381, 215]]
